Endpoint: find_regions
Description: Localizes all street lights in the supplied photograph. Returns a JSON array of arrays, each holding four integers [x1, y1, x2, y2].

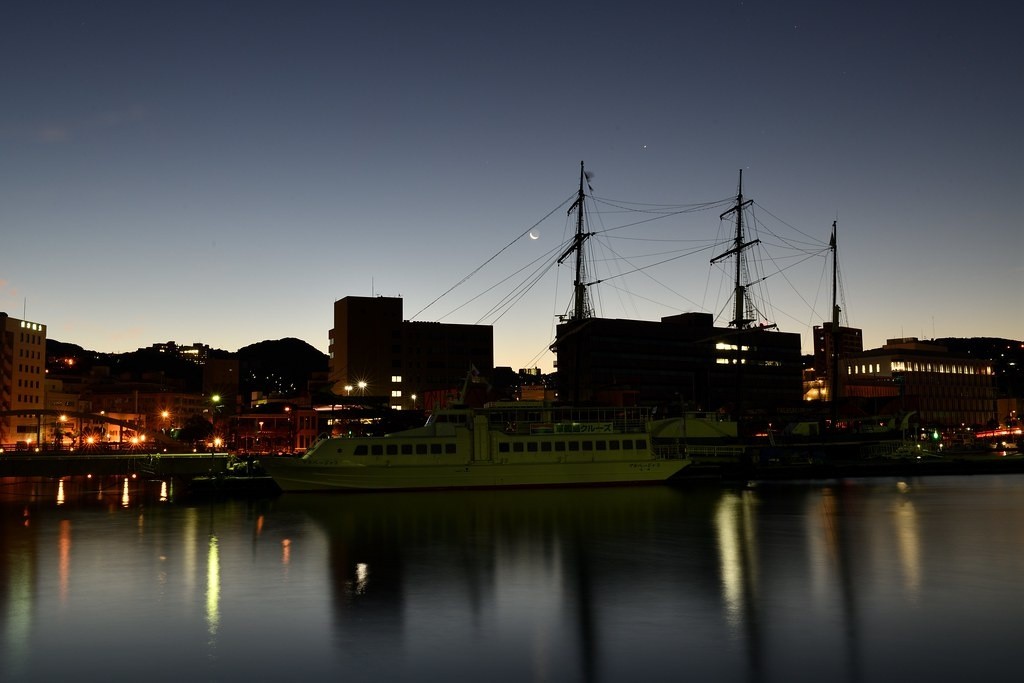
[[210, 394, 221, 454], [160, 410, 169, 438]]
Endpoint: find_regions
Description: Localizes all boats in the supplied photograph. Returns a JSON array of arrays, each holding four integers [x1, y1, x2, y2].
[[882, 445, 943, 462], [258, 402, 692, 490]]
